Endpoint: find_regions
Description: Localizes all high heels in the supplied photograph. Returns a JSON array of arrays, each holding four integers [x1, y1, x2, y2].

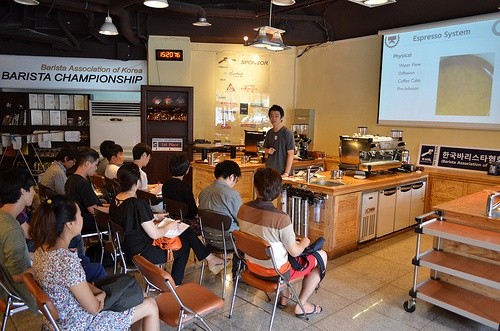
[[207, 256, 231, 274]]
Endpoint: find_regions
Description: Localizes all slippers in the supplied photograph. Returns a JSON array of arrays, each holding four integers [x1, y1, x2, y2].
[[277, 292, 293, 309], [295, 303, 322, 316]]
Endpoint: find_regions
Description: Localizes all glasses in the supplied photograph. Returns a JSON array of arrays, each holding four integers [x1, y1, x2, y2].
[[234, 178, 240, 183], [146, 156, 152, 159]]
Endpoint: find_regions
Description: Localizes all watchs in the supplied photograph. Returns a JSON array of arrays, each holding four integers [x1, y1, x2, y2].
[[284, 173, 290, 177]]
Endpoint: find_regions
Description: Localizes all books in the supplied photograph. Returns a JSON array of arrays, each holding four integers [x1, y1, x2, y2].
[[0, 93, 90, 174], [157, 217, 191, 239]]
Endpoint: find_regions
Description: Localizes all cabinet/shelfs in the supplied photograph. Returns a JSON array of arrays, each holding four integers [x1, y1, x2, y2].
[[0, 91, 89, 173]]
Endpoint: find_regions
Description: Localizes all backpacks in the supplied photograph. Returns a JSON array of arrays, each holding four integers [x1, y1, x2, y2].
[[288, 237, 326, 282]]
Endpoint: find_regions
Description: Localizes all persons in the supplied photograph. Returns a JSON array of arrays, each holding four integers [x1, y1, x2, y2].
[[98, 140, 115, 176], [161, 154, 198, 219], [110, 162, 233, 288], [236, 168, 327, 318], [104, 145, 124, 179], [0, 169, 105, 315], [199, 161, 245, 279], [259, 105, 295, 178], [32, 198, 161, 331], [64, 148, 117, 267], [130, 143, 160, 196], [41, 147, 79, 195]]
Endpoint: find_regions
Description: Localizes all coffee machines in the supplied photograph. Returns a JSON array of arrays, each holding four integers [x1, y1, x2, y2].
[[245, 130, 266, 164], [391, 129, 415, 173], [293, 133, 314, 161]]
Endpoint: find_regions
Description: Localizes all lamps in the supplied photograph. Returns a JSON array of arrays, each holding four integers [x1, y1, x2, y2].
[[98, 9, 119, 36], [192, 17, 212, 26], [143, 0, 169, 9], [244, 0, 295, 51]]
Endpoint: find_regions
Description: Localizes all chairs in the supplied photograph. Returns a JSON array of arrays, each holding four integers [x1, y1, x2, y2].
[[0, 175, 309, 331]]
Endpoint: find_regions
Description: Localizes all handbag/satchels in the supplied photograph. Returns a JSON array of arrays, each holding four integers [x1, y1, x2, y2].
[[94, 274, 144, 312], [86, 244, 114, 267], [152, 237, 182, 262]]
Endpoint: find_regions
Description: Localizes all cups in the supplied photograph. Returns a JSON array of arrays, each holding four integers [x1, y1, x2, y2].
[[331, 170, 343, 179], [240, 155, 247, 164]]
